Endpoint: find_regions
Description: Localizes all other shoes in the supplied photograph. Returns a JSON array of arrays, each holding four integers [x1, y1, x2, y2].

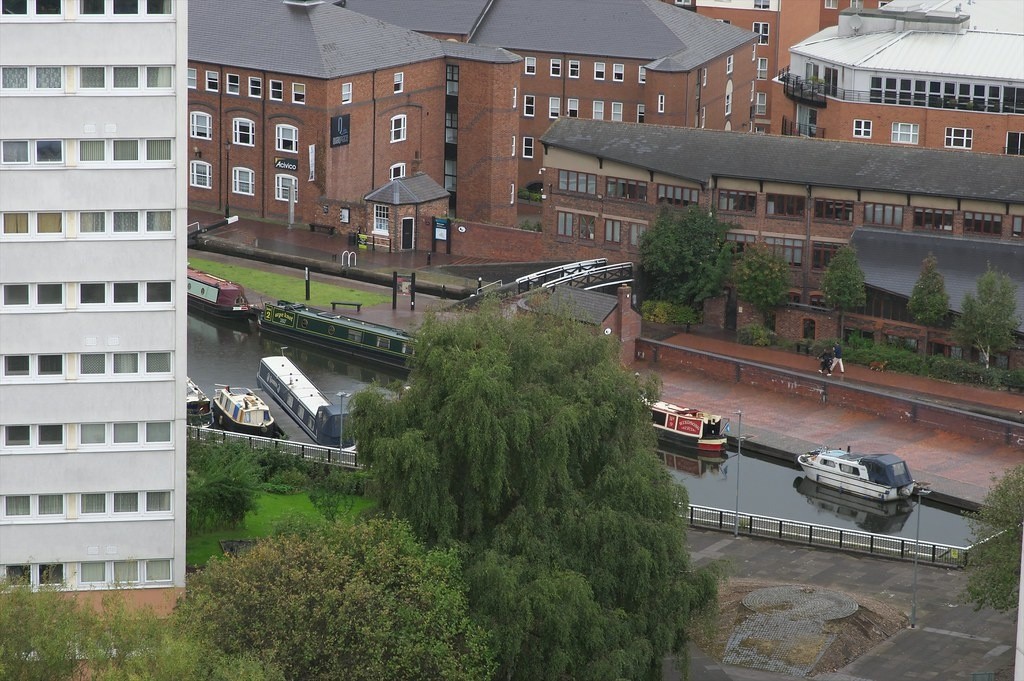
[[827, 374, 832, 376], [829, 370, 832, 372], [841, 371, 844, 373], [819, 369, 823, 374]]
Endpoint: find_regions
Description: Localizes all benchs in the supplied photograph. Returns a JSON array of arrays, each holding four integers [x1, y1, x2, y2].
[[309, 223, 335, 234], [331, 301, 362, 312]]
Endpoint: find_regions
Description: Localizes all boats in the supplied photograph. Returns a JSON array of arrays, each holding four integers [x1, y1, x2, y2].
[[186, 376, 213, 430], [210, 383, 275, 438], [258, 299, 430, 374], [187, 261, 258, 324], [795, 444, 932, 504], [257, 345, 357, 449], [640, 397, 730, 451]]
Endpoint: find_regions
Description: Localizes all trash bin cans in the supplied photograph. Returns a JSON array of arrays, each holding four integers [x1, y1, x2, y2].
[[347, 232, 356, 246]]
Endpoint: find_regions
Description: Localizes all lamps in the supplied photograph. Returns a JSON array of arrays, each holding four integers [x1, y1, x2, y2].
[[193, 146, 201, 158], [597, 192, 604, 203]]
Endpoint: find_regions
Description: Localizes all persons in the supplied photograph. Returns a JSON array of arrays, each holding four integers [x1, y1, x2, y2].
[[816, 347, 831, 377], [829, 340, 845, 373]]
